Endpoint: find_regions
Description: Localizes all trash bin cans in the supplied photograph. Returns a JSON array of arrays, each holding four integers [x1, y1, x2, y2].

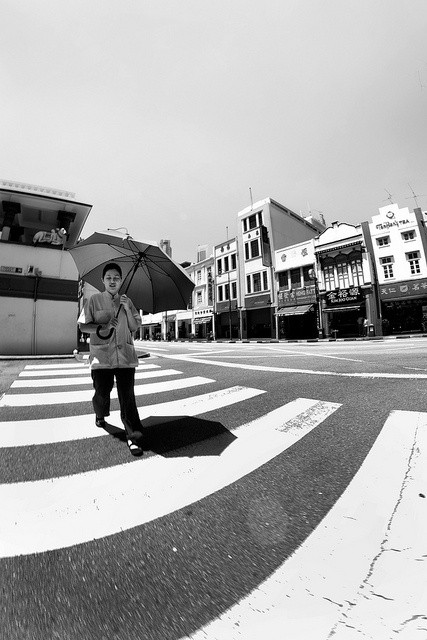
[[319, 328, 324, 339], [369, 324, 376, 337]]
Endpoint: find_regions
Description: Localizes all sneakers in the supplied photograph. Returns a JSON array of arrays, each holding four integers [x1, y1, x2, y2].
[[127, 439, 143, 456], [96, 415, 105, 427]]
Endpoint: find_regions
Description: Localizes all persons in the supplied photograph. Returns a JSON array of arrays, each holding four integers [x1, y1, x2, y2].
[[74, 262, 147, 459]]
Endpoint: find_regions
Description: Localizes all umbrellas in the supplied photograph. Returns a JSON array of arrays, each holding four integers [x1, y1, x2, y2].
[[67, 229, 196, 316]]
